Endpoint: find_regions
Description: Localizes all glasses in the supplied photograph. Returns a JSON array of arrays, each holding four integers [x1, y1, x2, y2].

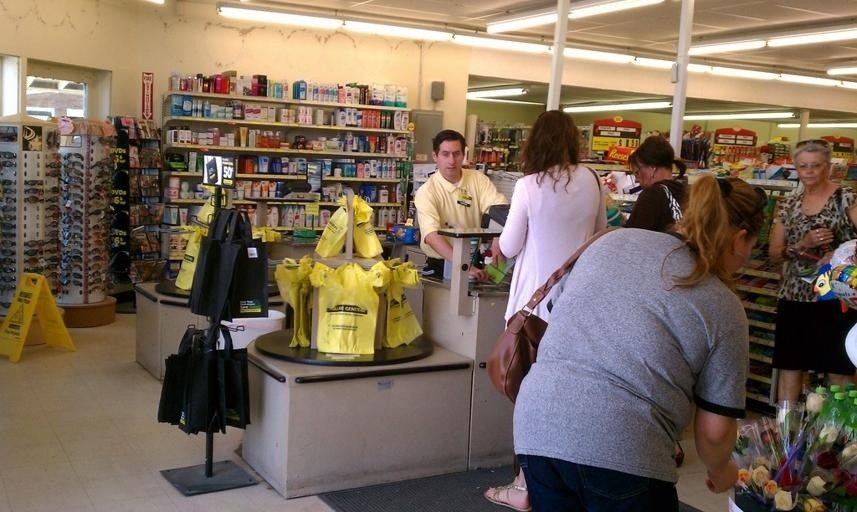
[[24, 180, 45, 203], [0, 151, 16, 308], [796, 140, 828, 149], [45, 153, 114, 294], [738, 187, 768, 228], [24, 240, 45, 273]]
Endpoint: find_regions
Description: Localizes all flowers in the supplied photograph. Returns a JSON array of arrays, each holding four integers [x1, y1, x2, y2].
[[727, 406, 857, 512]]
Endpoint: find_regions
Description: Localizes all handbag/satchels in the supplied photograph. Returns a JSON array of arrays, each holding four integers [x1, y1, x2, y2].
[[187, 208, 268, 323], [157, 325, 250, 435], [487, 309, 548, 405]]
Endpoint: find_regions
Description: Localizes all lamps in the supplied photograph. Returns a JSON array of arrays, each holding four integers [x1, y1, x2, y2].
[[215, 1, 856, 89]]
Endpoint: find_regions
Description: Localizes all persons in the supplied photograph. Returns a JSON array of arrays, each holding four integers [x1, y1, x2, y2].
[[484, 110, 608, 512], [512, 174, 769, 512], [414, 129, 509, 283], [768, 141, 857, 411], [620, 136, 693, 236]]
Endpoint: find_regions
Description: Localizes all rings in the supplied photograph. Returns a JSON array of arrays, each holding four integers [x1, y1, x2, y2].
[[815, 229, 820, 233], [817, 233, 821, 237], [820, 237, 824, 241]]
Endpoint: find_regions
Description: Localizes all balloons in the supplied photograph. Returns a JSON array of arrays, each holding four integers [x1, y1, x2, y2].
[[811, 240, 857, 312]]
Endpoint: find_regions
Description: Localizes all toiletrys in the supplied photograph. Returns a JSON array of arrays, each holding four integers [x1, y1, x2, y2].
[[166, 70, 409, 230]]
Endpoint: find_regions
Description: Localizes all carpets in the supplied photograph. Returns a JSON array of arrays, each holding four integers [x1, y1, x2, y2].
[[318, 462, 702, 511]]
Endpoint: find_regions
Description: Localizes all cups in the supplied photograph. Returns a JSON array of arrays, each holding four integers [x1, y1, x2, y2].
[[607, 208, 625, 228]]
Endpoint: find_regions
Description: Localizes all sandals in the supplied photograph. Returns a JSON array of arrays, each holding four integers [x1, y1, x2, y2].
[[483, 485, 533, 512]]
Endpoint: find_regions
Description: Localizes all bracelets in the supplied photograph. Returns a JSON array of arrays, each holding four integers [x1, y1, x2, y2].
[[781, 246, 792, 261]]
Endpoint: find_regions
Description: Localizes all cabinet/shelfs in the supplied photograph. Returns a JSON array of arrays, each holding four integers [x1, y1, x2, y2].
[[1, 91, 857, 500]]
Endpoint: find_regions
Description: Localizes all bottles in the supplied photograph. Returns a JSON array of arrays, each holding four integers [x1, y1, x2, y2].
[[816, 382, 857, 437]]
